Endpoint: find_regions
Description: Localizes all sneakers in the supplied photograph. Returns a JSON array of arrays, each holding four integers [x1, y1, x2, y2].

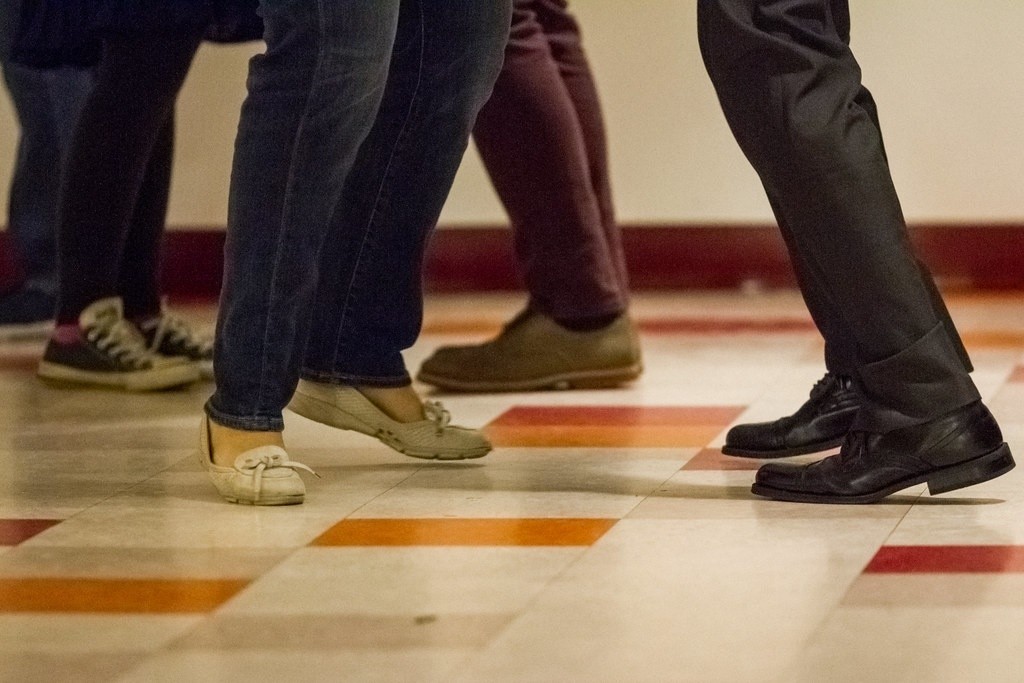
[[419, 306, 643, 394], [39, 307, 218, 388]]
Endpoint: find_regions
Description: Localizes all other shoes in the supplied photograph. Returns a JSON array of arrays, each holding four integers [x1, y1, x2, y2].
[[198, 412, 309, 507], [289, 374, 495, 461]]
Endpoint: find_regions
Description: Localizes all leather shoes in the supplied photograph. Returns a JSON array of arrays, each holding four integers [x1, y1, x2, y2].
[[720, 373, 1015, 503]]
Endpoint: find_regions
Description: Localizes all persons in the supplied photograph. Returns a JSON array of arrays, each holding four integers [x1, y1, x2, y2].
[[696, 0, 1015, 503], [0, 0, 645, 507]]
